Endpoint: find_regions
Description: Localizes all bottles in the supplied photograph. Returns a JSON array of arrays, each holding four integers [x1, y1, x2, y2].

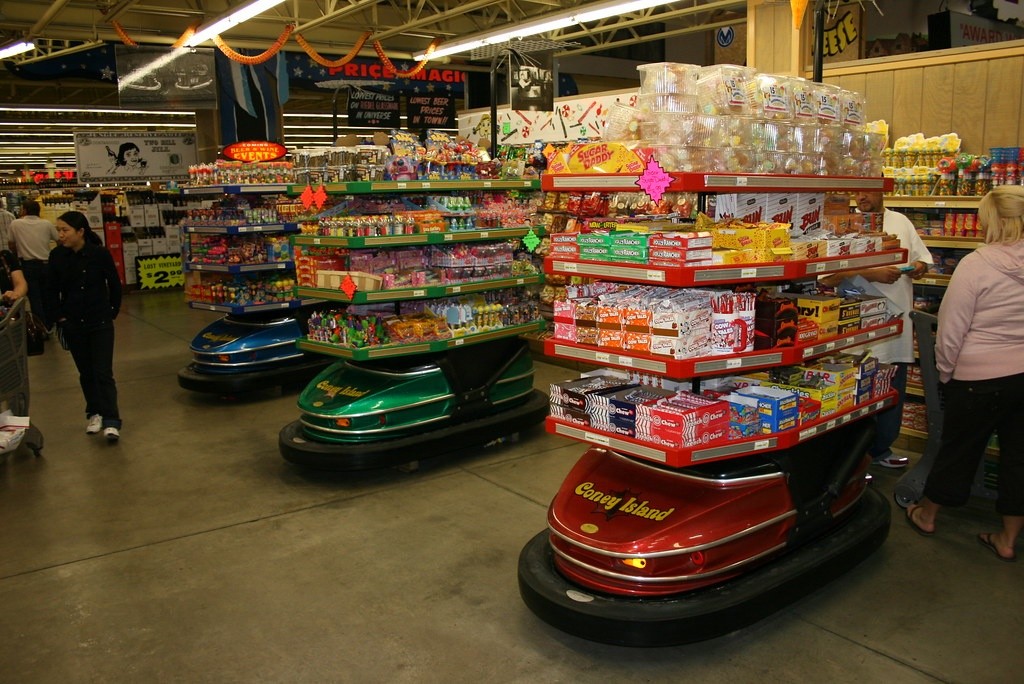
[[128, 196, 186, 225], [101, 196, 128, 227], [38, 188, 100, 225], [187, 162, 294, 185], [122, 235, 165, 244], [0, 177, 78, 190], [105, 146, 120, 166]]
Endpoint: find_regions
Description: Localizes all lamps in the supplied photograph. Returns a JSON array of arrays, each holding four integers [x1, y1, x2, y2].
[[118, 0, 285, 91], [412, 1, 677, 62], [0, 38, 38, 59]]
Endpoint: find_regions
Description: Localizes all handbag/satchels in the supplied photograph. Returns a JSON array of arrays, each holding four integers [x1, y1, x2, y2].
[[25, 312, 45, 357], [55, 324, 68, 351]]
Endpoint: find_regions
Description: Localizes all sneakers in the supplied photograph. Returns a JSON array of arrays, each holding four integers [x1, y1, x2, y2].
[[872, 452, 909, 468], [104, 427, 119, 441], [87, 414, 103, 433]]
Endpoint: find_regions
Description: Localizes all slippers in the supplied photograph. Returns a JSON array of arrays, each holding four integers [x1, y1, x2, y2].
[[976, 533, 1016, 562], [905, 504, 935, 536]]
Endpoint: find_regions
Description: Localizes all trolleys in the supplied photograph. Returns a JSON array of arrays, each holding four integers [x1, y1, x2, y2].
[[0, 294, 44, 468]]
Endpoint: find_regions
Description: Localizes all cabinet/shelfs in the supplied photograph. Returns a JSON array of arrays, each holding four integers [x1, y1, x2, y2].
[[181, 185, 330, 313], [285, 177, 548, 360], [885, 195, 1000, 456], [540, 173, 900, 471]]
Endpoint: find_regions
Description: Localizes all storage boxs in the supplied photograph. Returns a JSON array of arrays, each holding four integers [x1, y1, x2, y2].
[[102, 190, 181, 286], [298, 63, 900, 446]]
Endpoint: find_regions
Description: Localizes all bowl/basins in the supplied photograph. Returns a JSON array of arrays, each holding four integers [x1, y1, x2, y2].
[[79, 176, 146, 182]]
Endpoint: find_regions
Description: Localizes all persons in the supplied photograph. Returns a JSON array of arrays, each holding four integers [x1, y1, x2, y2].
[[905, 185, 1024, 562], [817, 191, 935, 467], [0, 198, 16, 251], [45, 210, 123, 439], [0, 249, 28, 307], [8, 201, 62, 339]]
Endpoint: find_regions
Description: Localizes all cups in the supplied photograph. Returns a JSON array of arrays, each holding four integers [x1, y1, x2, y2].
[[710, 309, 755, 354]]
